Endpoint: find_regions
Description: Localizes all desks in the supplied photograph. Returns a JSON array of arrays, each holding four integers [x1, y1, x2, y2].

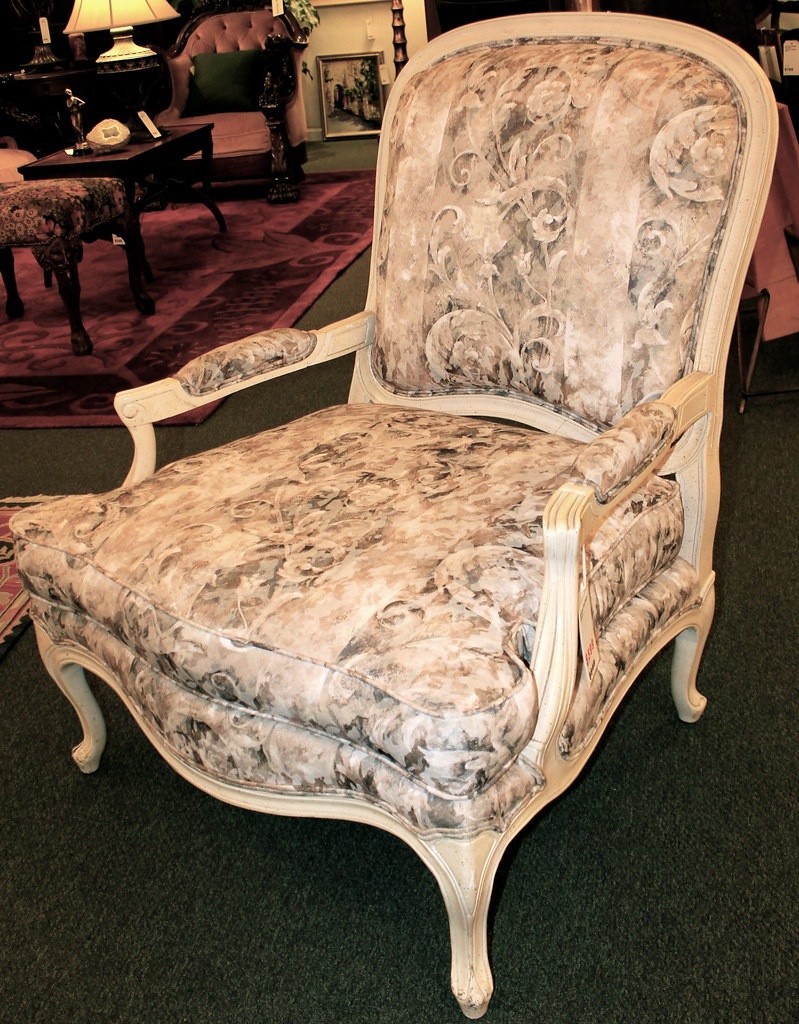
[[0, 65, 96, 153], [17, 123, 227, 288]]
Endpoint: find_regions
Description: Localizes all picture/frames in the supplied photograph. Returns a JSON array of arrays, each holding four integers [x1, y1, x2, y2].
[[315, 51, 384, 143]]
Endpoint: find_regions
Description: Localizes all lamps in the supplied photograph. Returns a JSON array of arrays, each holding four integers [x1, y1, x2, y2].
[[63, 0, 180, 75]]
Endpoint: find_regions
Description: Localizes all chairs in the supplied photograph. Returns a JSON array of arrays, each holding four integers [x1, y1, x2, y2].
[[9, 12, 780, 1019], [137, 5, 309, 210]]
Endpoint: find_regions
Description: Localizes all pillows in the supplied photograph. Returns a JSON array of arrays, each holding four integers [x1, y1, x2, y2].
[[179, 51, 264, 117]]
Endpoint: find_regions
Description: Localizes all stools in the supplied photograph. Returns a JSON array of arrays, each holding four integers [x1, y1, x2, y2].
[[0, 177, 156, 355]]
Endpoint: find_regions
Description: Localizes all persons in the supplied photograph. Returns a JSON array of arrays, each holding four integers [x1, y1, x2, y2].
[[65, 89, 86, 143]]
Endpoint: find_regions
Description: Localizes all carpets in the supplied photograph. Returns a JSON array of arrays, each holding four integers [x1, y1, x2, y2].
[[0, 168, 375, 428]]
